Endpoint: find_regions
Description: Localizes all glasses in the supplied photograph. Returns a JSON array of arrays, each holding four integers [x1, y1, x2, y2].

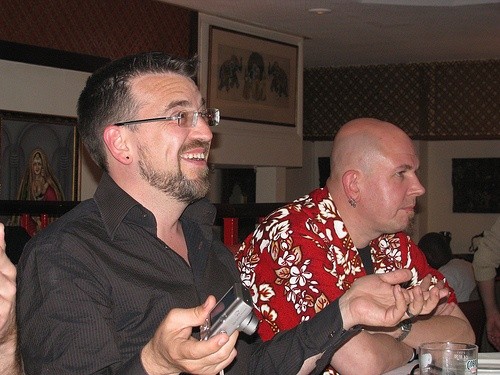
[[113, 108, 220, 128]]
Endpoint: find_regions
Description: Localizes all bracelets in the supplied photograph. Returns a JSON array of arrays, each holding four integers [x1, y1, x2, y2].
[[408, 348, 416, 362]]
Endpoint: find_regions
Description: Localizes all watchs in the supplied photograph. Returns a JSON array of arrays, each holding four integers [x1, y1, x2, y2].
[[396, 313, 413, 342]]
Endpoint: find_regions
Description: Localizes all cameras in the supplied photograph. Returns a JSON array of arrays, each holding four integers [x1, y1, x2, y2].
[[200, 281, 260, 342]]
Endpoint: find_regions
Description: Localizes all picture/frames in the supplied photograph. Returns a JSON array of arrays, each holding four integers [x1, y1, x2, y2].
[[207, 25, 299, 128], [0, 109, 84, 217]]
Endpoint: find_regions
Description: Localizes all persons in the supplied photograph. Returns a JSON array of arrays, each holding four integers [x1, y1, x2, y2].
[[14, 56, 452, 375], [418, 232, 484, 352], [472, 214, 500, 353], [0, 223, 23, 375], [234, 118, 476, 375]]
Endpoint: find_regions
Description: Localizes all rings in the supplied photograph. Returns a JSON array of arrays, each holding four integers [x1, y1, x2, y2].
[[406, 302, 414, 318]]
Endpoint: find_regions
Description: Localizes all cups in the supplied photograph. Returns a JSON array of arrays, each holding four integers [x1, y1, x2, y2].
[[418, 341, 478, 375]]
